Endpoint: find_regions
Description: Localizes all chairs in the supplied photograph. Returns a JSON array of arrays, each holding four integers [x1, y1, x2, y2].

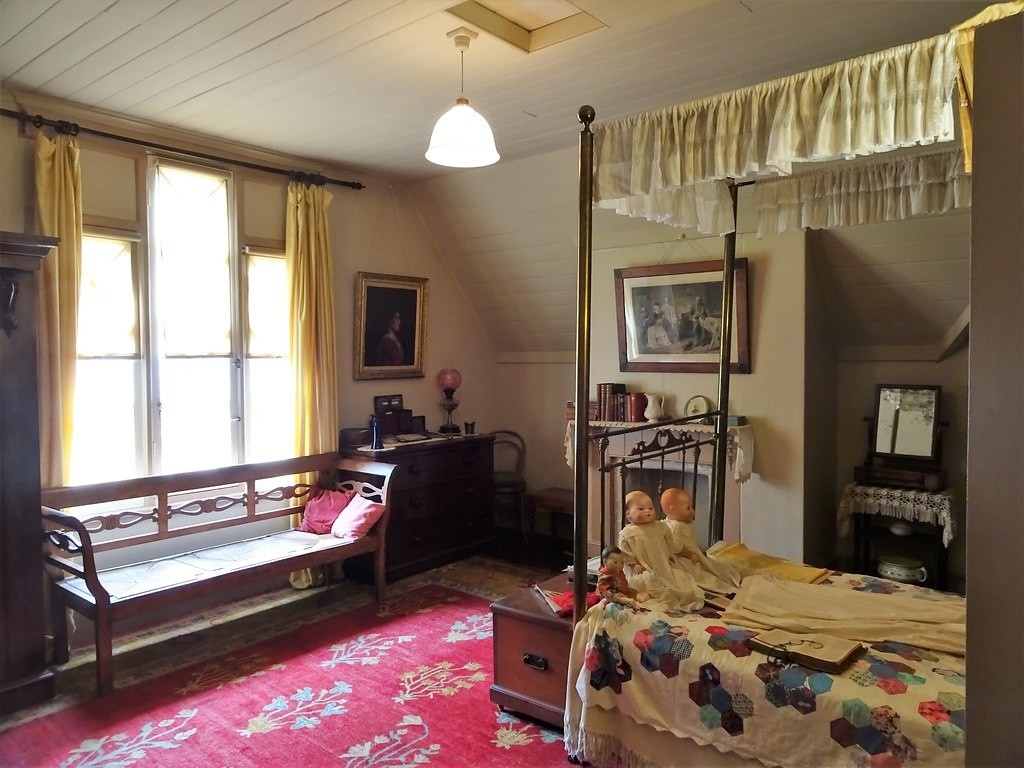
[[487, 430, 527, 546]]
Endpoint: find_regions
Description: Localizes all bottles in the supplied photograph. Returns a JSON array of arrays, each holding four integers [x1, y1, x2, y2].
[[368, 414, 385, 449]]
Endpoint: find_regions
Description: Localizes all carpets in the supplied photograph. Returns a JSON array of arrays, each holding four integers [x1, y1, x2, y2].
[[0, 583, 634, 768]]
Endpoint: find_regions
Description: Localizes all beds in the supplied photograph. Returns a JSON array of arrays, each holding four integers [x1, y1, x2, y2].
[[562, 0, 1024, 768]]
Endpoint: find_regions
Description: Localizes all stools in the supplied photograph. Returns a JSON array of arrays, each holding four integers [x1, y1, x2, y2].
[[524, 486, 574, 545]]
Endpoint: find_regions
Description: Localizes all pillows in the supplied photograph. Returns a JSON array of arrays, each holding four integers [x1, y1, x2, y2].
[[294, 480, 357, 535], [331, 492, 386, 541]]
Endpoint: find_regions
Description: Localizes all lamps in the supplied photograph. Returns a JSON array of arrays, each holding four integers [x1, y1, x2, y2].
[[438, 368, 461, 433], [425, 27, 500, 169]]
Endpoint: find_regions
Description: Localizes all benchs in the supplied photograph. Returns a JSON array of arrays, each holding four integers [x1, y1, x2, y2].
[[490, 555, 602, 728], [40, 451, 400, 699]]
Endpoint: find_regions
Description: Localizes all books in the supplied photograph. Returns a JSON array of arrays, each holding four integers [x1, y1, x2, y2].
[[565, 383, 648, 423], [747, 629, 863, 674]]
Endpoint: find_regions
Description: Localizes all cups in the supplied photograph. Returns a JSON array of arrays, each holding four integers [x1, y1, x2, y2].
[[465, 421, 475, 435]]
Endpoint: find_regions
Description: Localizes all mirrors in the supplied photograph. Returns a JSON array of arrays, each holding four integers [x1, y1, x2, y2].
[[871, 383, 943, 461]]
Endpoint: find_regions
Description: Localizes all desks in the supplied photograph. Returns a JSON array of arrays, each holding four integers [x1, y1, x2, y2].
[[337, 427, 497, 587], [837, 482, 957, 593]]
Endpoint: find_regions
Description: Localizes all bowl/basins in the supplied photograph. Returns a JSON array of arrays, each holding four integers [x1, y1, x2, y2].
[[890, 521, 914, 536], [878, 555, 927, 583], [341, 428, 370, 444]]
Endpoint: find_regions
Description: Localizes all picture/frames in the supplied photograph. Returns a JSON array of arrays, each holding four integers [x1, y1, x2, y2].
[[353, 270, 429, 381], [614, 257, 751, 373]]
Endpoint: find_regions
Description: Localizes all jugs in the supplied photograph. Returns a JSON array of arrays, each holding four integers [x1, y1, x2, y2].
[[644, 393, 664, 423]]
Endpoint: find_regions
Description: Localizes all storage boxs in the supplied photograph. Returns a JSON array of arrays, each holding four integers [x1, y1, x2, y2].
[[726, 415, 746, 427]]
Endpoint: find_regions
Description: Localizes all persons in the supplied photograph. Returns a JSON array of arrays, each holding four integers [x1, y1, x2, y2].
[[619, 490, 705, 613], [597, 545, 650, 609], [660, 488, 707, 580]]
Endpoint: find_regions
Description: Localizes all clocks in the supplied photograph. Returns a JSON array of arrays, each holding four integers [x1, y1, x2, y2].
[[683, 395, 709, 422]]
